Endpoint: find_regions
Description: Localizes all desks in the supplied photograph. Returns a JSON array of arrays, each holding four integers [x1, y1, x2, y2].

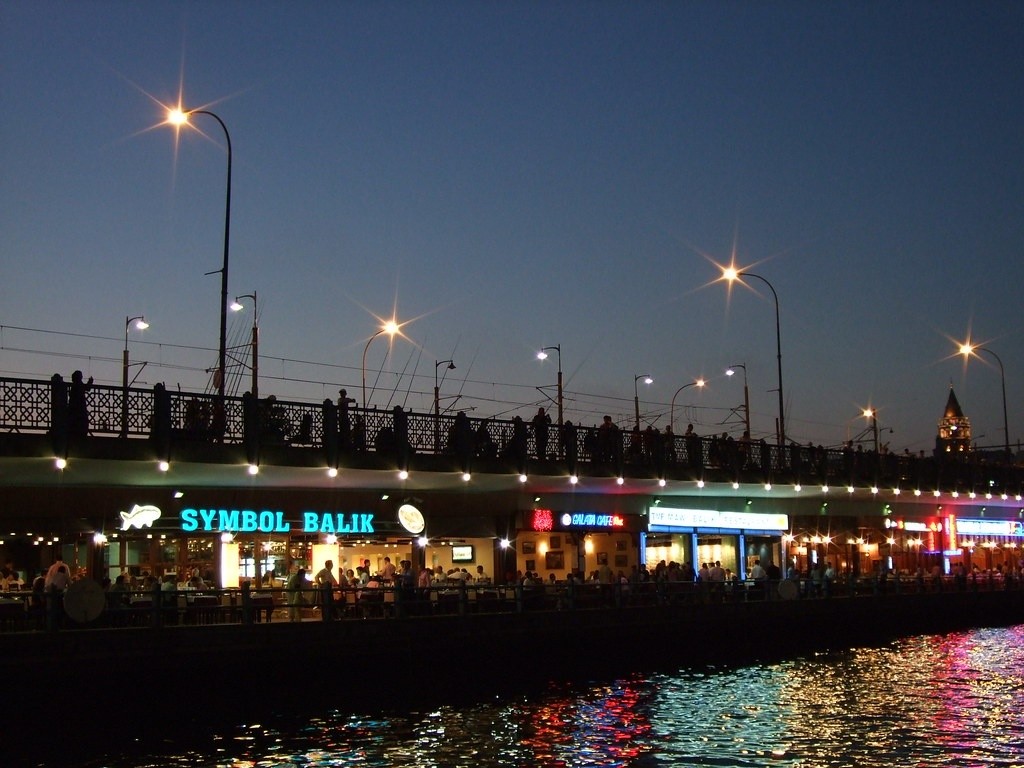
[[178, 596, 218, 625], [332, 593, 395, 618], [131, 597, 153, 623], [431, 590, 468, 604], [0, 599, 24, 632], [222, 595, 273, 623], [469, 590, 499, 600]]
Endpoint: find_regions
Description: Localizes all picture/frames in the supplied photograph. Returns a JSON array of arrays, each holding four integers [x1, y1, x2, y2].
[[526, 560, 535, 570], [617, 540, 627, 551], [522, 542, 536, 554], [545, 551, 564, 570], [597, 552, 608, 565], [550, 536, 560, 548], [614, 555, 628, 567]]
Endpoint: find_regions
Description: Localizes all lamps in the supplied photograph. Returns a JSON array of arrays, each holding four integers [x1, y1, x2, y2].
[[885, 503, 890, 509], [744, 498, 753, 505], [1020, 508, 1024, 513], [822, 500, 828, 507], [653, 496, 661, 504], [937, 504, 942, 510], [534, 495, 542, 502]]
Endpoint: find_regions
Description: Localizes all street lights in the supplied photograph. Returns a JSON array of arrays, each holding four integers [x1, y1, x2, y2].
[[123, 317, 149, 451], [961, 345, 1010, 462], [363, 323, 397, 459], [727, 363, 753, 464], [877, 427, 895, 464], [724, 269, 786, 473], [539, 345, 565, 461], [230, 295, 260, 463], [862, 407, 879, 472], [672, 381, 705, 442], [170, 109, 232, 458], [434, 360, 457, 456], [634, 374, 653, 434]]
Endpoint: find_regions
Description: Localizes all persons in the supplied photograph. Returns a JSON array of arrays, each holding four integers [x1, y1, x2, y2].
[[62, 371, 1024, 499], [1, 556, 1024, 624]]
